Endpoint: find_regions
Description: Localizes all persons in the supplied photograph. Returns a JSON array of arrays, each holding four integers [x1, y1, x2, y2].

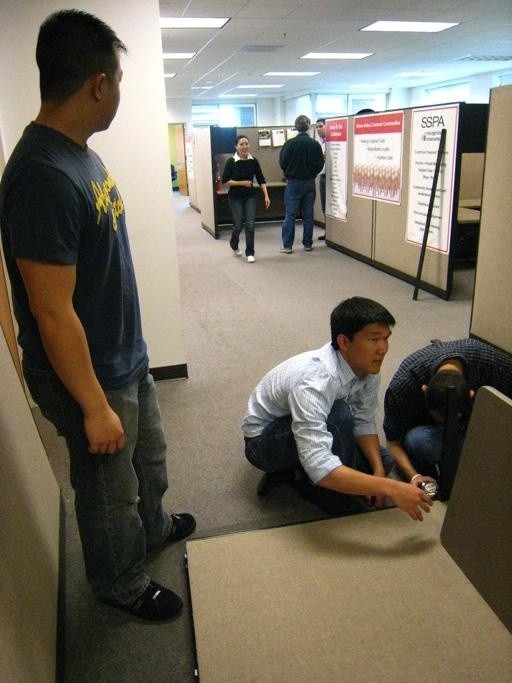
[[221, 135, 271, 264], [279, 115, 325, 254], [241, 297, 434, 522], [0, 9, 197, 623], [383, 337, 511, 502]]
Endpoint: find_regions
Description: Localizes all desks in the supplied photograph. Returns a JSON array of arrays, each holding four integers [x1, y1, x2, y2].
[[215, 180, 286, 195], [456, 198, 481, 225]]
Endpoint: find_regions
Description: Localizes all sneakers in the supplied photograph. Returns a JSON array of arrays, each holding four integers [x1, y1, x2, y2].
[[148, 511, 197, 551], [234, 246, 255, 263], [304, 244, 312, 251], [256, 469, 289, 498], [97, 580, 184, 625], [279, 246, 292, 253]]
